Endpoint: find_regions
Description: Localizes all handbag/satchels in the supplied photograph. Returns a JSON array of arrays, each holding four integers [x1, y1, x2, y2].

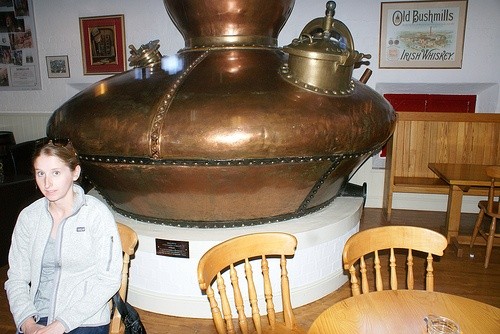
[[110, 292, 147, 334]]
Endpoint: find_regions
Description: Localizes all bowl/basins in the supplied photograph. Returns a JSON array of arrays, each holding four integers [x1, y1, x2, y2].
[[426, 313, 459, 334]]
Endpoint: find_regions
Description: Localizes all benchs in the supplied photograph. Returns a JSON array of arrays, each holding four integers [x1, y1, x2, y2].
[[384, 112, 500, 223]]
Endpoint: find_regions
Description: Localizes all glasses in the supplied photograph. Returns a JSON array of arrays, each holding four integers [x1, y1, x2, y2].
[[35, 137, 69, 152]]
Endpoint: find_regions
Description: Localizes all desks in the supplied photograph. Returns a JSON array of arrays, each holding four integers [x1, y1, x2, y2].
[[306, 290, 500, 334], [427, 165, 500, 257]]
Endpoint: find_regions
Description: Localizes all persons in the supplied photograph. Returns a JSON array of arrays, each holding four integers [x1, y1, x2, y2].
[[4, 137, 124, 334]]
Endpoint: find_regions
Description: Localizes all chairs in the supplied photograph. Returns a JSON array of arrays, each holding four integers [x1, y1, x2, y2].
[[470, 166, 500, 268], [108, 222, 138, 334], [197, 232, 307, 334], [343, 226, 447, 296], [0, 131, 37, 177]]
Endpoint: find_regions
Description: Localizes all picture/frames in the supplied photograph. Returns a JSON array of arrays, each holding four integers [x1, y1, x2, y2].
[[79, 14, 127, 75], [379, 0, 470, 69], [46, 55, 70, 78]]
